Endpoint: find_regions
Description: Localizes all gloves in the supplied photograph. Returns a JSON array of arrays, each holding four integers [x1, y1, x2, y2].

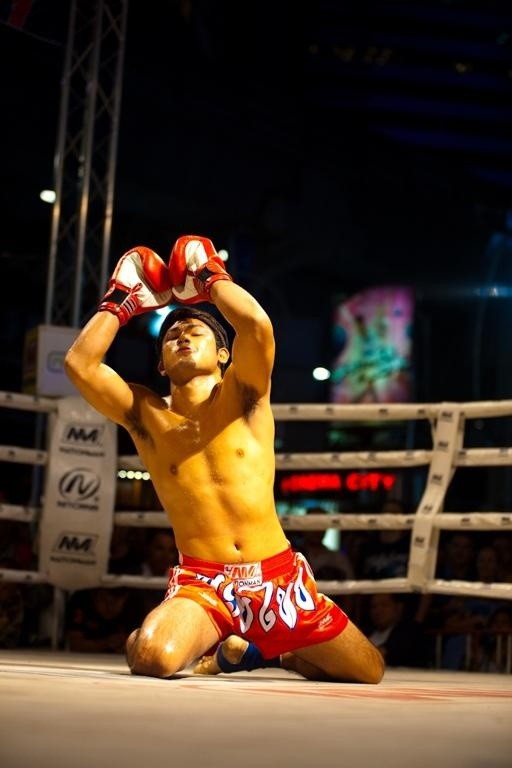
[[96, 243, 174, 328], [167, 232, 235, 305]]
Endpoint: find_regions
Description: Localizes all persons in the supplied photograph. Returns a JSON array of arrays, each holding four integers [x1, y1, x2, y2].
[[59, 230, 388, 687]]
